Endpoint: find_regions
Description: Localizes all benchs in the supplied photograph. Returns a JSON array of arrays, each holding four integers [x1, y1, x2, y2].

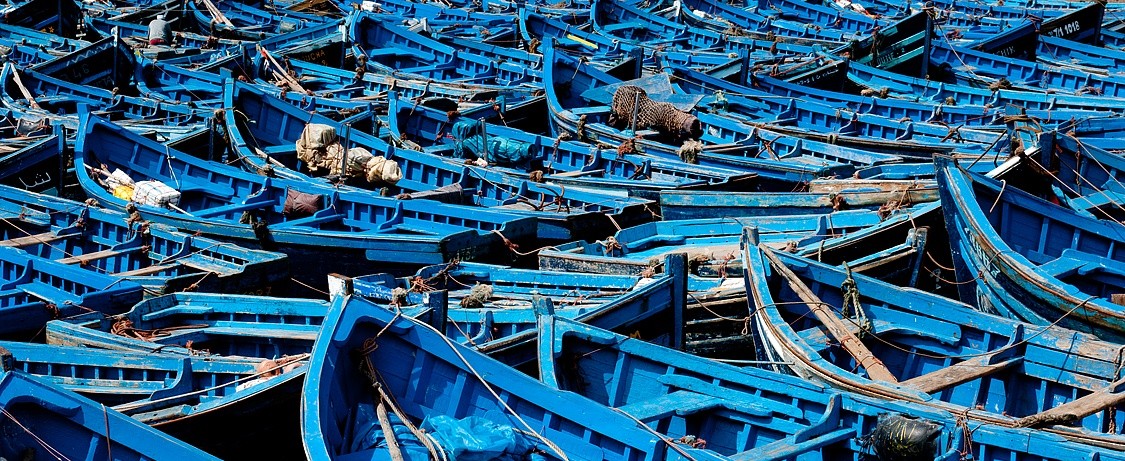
[[826, 130, 858, 136], [1071, 190, 1122, 210], [612, 391, 718, 425], [0, 94, 126, 299], [362, 225, 397, 234], [1033, 383, 1125, 423], [1037, 256, 1087, 279], [751, 116, 798, 126], [268, 211, 345, 228], [898, 345, 1028, 395], [549, 166, 606, 179], [700, 142, 758, 153], [141, 0, 824, 106], [570, 105, 611, 115], [796, 316, 897, 355], [403, 141, 476, 200], [261, 142, 300, 157], [635, 129, 660, 137], [165, 181, 274, 218], [728, 426, 857, 461]]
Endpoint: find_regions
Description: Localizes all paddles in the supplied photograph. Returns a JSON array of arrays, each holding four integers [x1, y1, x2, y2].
[[261, 45, 307, 93], [10, 62, 42, 110], [756, 240, 898, 384], [203, 0, 235, 27]]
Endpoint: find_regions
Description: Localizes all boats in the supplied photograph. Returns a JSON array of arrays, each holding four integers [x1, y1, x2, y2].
[[738, 224, 1125, 451], [0, 340, 310, 435], [932, 152, 1125, 342], [1037, 129, 1125, 221], [0, 1, 1125, 370], [530, 292, 970, 461], [0, 369, 224, 461], [298, 293, 737, 461]]
